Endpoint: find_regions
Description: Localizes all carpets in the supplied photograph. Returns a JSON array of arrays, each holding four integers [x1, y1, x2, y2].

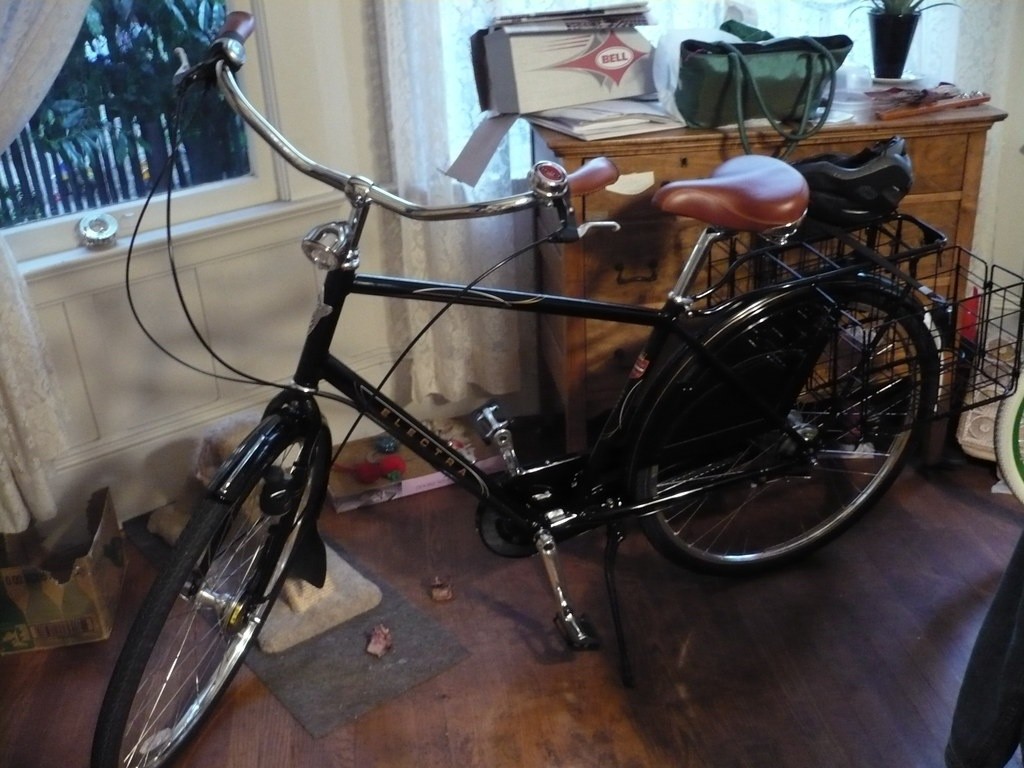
[[118, 497, 475, 741]]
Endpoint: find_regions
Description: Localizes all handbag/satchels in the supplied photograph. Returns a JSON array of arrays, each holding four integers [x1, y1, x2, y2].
[[675, 34, 853, 161]]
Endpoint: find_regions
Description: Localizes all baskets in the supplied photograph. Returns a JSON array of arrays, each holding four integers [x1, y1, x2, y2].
[[707, 219, 1024, 444]]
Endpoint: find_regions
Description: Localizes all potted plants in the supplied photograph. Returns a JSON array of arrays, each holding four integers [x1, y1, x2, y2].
[[848, 0, 964, 83]]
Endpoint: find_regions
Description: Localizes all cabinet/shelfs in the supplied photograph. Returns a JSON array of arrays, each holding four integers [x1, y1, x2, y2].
[[524, 76, 1007, 489]]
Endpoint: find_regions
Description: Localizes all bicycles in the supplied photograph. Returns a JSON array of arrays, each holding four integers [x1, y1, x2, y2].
[[88, 13, 1024, 768]]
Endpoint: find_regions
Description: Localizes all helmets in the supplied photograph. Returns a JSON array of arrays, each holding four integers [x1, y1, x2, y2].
[[793, 136, 914, 227]]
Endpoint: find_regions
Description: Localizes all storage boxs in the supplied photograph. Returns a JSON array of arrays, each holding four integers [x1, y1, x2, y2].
[[0, 489, 130, 652]]
